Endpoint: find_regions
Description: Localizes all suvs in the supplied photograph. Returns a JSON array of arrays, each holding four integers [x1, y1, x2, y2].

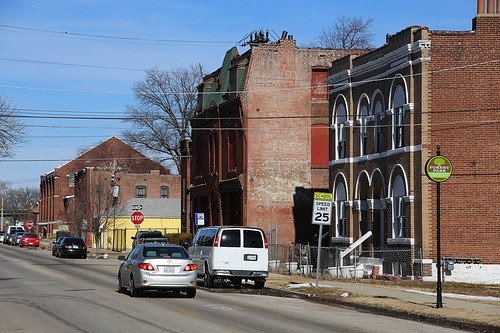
[[184, 225, 269, 289]]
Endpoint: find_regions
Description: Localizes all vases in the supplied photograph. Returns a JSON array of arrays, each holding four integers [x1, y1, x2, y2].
[[327, 266, 365, 279], [269, 260, 280, 269], [285, 262, 298, 271], [300, 265, 313, 274]]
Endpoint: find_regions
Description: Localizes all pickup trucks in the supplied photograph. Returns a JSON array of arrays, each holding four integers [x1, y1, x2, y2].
[[130, 230, 169, 250]]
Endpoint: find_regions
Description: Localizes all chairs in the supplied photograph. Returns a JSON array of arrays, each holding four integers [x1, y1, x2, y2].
[[147, 252, 156, 257], [172, 253, 181, 257]]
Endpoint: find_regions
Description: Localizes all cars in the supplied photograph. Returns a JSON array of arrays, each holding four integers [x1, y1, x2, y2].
[[118, 243, 197, 298], [52, 237, 69, 257], [56, 237, 87, 259], [0, 226, 40, 248]]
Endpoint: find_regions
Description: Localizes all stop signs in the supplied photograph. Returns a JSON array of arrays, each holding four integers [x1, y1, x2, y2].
[[26, 220, 34, 228], [130, 212, 145, 224]]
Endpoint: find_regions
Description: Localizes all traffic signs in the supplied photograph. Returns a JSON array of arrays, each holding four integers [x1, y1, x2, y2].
[[131, 205, 142, 211]]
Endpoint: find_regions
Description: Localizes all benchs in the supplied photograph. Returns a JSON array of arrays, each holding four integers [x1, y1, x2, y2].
[[354, 257, 383, 278]]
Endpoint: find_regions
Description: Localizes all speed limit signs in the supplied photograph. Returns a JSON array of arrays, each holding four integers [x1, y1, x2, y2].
[[311, 191, 333, 226]]
